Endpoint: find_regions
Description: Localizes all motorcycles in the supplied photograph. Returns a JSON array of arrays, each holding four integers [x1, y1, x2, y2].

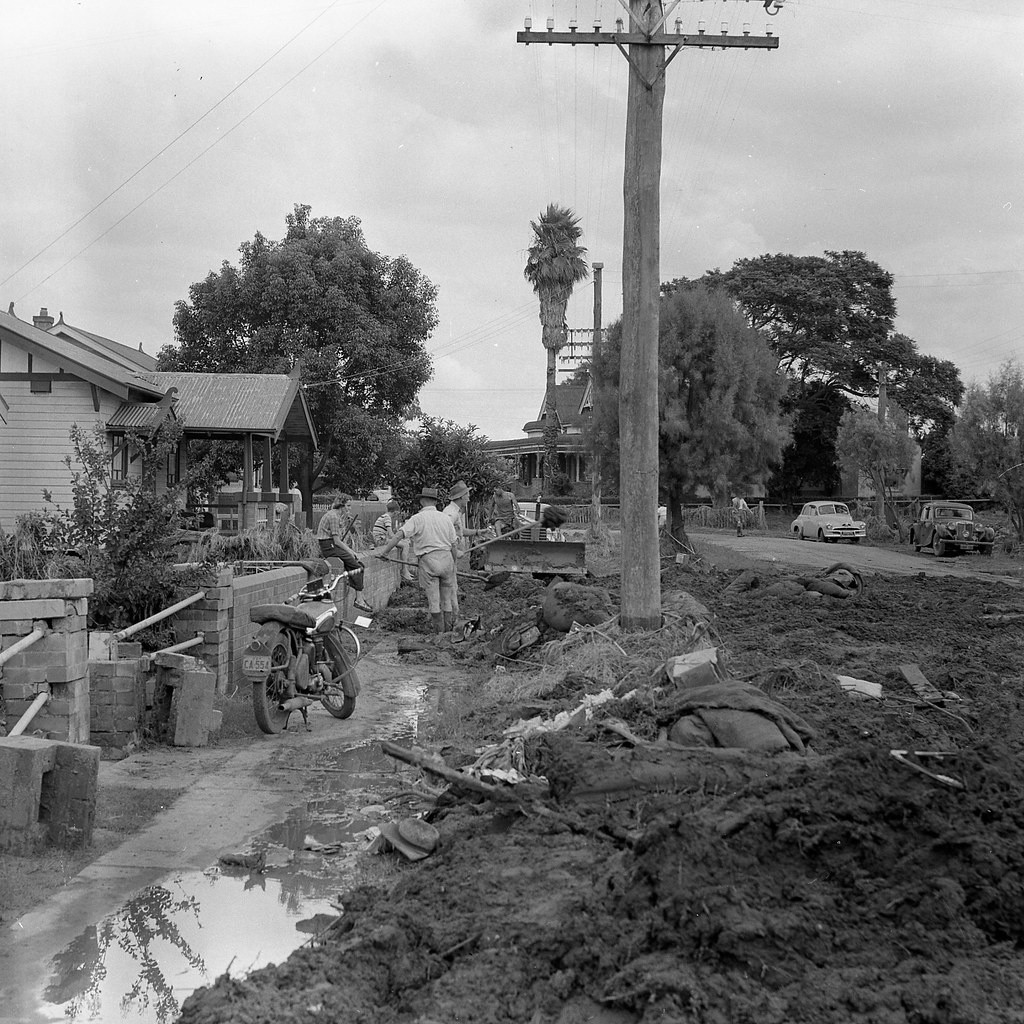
[[245, 567, 367, 733]]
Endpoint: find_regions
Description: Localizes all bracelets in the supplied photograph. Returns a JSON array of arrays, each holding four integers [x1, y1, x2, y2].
[[476, 529, 478, 535]]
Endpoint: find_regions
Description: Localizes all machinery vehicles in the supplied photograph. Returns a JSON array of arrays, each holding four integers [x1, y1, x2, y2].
[[470, 495, 587, 579]]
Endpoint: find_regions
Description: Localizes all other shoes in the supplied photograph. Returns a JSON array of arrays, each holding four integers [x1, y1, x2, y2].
[[354, 597, 374, 613]]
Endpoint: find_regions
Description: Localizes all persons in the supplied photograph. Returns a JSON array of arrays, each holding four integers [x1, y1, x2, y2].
[[380, 488, 457, 635], [681, 504, 686, 528], [658, 502, 667, 536], [443, 481, 492, 595], [373, 502, 414, 581], [317, 493, 374, 612], [732, 491, 753, 537], [485, 486, 520, 537]]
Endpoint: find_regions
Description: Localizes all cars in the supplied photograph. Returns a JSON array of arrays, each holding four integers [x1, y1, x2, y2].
[[910, 502, 996, 559], [789, 501, 868, 543]]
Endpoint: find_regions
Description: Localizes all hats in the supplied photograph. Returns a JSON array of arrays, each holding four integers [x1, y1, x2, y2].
[[415, 488, 441, 502], [447, 480, 473, 500]]
